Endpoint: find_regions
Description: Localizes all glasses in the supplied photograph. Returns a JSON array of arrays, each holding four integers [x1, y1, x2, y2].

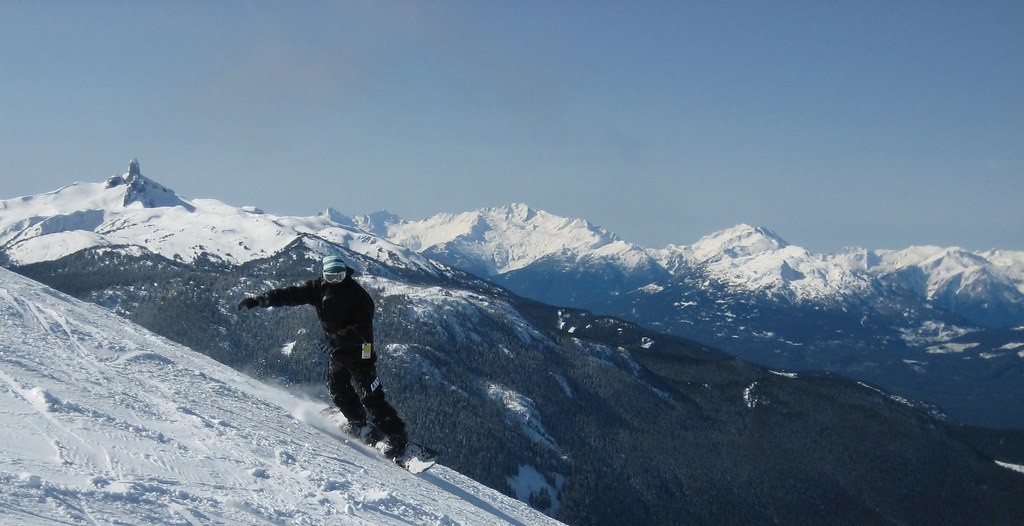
[[323, 269, 346, 283]]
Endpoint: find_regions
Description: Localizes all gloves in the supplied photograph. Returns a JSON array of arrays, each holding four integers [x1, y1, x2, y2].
[[238, 297, 259, 311], [318, 335, 340, 357]]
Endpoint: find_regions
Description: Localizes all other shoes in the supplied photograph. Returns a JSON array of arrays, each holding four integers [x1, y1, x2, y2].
[[384, 428, 410, 458], [342, 413, 366, 439]]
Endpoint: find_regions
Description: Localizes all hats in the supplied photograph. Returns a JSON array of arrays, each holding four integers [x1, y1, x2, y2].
[[322, 253, 346, 273]]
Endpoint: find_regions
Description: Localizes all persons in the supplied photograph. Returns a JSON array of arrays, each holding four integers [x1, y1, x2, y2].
[[238, 255, 407, 459]]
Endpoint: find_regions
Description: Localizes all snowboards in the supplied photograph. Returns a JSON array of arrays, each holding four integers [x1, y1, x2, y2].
[[315, 403, 440, 475]]
